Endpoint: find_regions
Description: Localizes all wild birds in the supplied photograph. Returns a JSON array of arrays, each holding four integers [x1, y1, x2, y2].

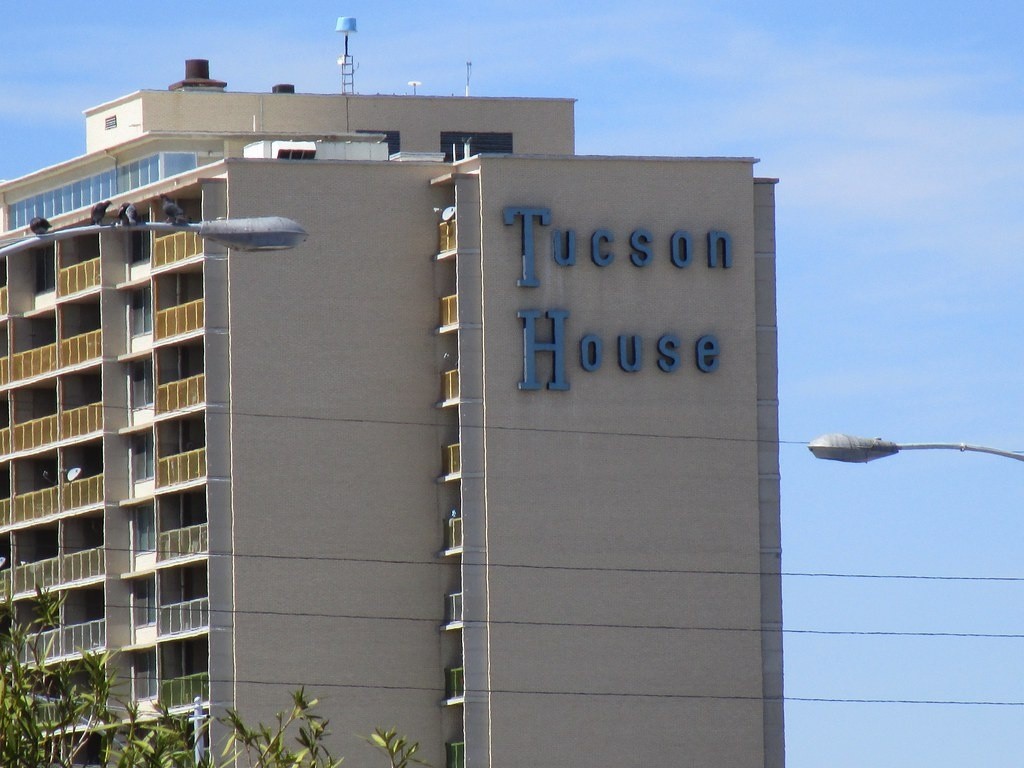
[[29, 216, 52, 235], [90, 201, 138, 226], [158, 193, 183, 225]]
